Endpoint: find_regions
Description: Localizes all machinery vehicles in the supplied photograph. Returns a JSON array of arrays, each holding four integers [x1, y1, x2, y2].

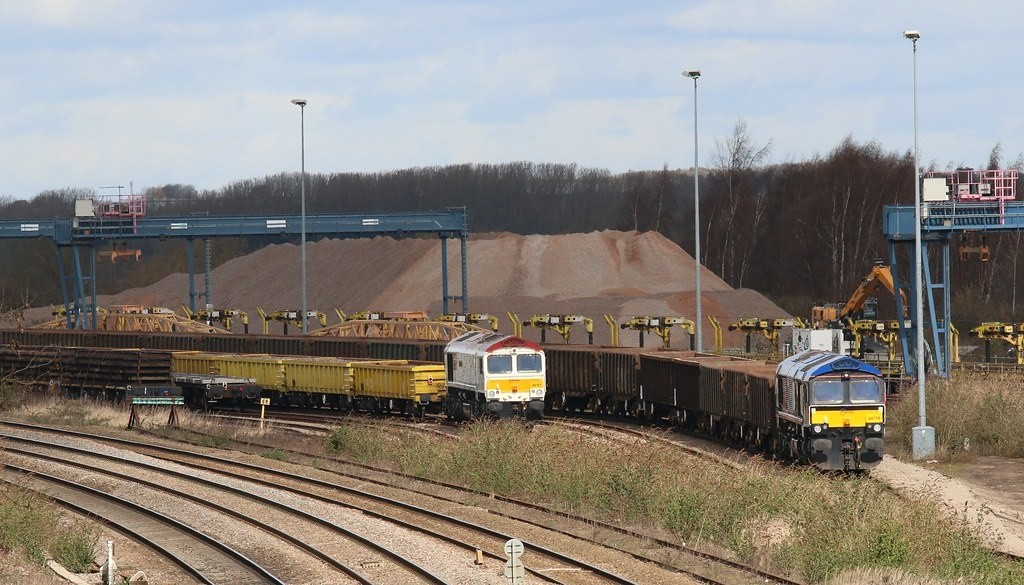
[[811, 265, 908, 329]]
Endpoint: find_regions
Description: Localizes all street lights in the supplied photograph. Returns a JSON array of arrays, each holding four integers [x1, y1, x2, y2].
[[291, 99, 307, 334], [904, 29, 926, 424], [682, 69, 702, 352]]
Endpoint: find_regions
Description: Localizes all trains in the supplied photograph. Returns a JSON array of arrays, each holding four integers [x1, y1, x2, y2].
[[0, 329, 888, 478]]
